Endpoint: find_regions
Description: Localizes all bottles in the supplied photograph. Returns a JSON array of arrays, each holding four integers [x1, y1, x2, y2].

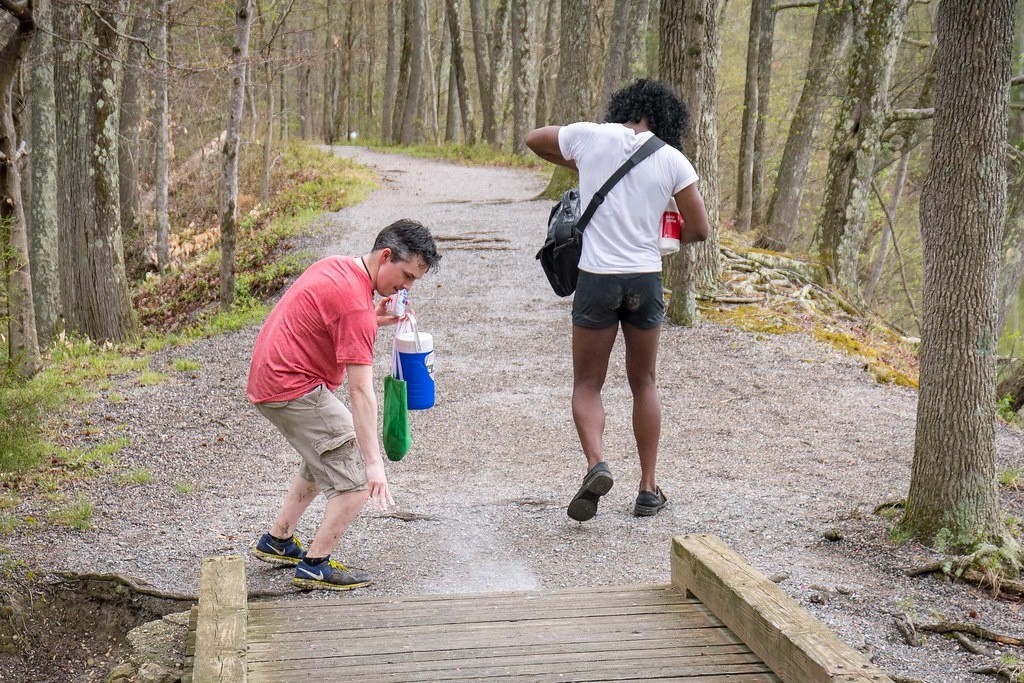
[[659, 197, 681, 255]]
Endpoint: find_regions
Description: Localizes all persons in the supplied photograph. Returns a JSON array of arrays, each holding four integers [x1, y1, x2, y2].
[[526, 77, 709, 521], [247, 218, 442, 591]]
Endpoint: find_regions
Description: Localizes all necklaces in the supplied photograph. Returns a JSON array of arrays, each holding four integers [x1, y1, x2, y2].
[[360, 257, 375, 296]]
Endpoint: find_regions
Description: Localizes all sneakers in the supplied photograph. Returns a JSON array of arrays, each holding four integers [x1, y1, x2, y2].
[[254, 531, 308, 565], [292, 552, 373, 591]]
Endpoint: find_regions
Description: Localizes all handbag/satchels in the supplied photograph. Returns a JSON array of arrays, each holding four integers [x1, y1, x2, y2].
[[383, 315, 410, 461], [536, 188, 582, 297]]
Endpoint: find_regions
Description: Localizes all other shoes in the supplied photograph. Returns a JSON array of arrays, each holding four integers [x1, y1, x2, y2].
[[567, 461, 613, 521], [635, 484, 670, 516]]
